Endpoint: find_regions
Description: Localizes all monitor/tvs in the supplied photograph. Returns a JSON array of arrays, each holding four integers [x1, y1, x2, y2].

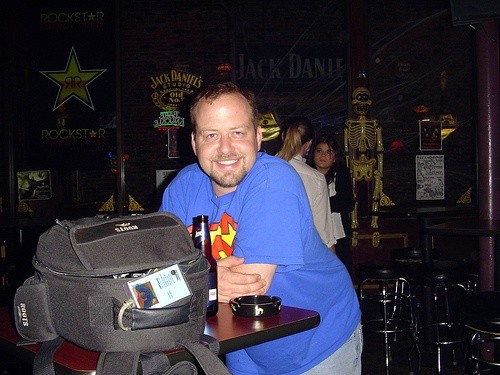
[[17, 170, 52, 202]]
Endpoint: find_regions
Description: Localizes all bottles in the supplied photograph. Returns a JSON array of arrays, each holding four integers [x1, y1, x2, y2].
[[194, 215, 219, 318]]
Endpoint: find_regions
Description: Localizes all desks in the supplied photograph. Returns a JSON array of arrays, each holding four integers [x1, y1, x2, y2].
[[0, 303, 321, 375], [368, 206, 478, 369], [0, 217, 49, 285], [420, 220, 500, 375]]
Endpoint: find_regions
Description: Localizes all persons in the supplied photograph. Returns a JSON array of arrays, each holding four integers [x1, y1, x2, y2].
[[273, 116, 337, 255], [157, 81, 363, 375], [305, 133, 355, 277]]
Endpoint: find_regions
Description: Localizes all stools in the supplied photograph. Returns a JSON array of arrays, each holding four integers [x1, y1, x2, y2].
[[355, 247, 500, 375]]
